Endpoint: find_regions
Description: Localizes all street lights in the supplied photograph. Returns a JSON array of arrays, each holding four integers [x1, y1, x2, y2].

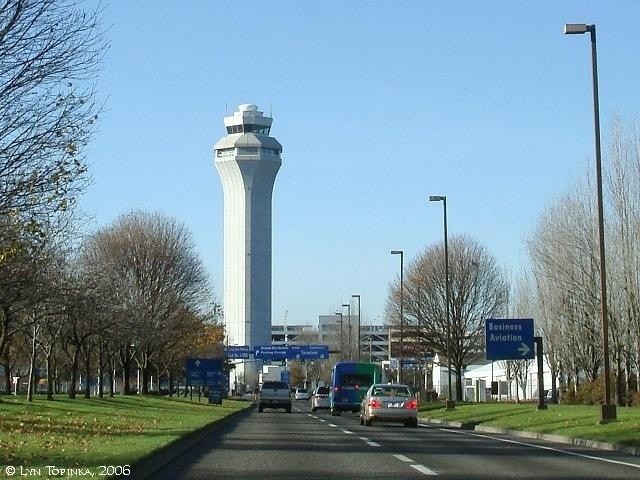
[[389, 250, 404, 385], [335, 294, 361, 363], [562, 22, 621, 425], [429, 195, 460, 412]]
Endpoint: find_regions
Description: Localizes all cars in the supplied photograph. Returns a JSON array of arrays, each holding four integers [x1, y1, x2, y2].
[[256, 363, 419, 429]]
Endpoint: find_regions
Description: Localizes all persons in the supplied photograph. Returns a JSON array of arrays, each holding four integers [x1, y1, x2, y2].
[[253, 384, 261, 401]]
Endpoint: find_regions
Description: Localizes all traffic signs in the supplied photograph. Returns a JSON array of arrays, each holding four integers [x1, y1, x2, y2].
[[290, 345, 330, 359], [485, 318, 535, 360], [224, 346, 249, 359], [253, 345, 290, 360], [186, 360, 223, 387]]
